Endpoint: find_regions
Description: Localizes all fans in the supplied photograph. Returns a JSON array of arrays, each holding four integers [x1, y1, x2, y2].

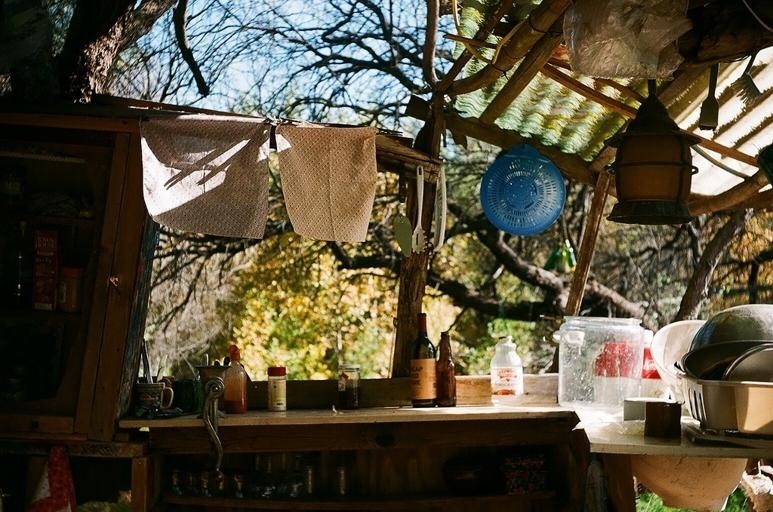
[[480, 142, 568, 236]]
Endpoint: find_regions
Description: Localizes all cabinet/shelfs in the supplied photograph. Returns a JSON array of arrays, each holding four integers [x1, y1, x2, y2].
[[0, 115, 154, 442]]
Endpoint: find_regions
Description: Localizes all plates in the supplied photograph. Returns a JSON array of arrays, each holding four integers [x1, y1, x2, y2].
[[623, 396, 683, 422]]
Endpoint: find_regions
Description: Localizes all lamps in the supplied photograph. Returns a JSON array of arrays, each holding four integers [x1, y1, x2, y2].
[[604, 77, 702, 229]]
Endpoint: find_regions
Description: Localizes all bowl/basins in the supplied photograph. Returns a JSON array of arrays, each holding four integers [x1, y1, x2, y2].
[[650, 303, 760, 396]]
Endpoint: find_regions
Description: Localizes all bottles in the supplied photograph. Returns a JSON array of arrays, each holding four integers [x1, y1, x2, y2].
[[436, 332, 456, 408], [223, 344, 247, 411], [489, 336, 523, 407], [409, 314, 436, 408], [267, 367, 287, 412]]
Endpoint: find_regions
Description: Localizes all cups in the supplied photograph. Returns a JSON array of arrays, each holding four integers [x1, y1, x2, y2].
[[135, 382, 172, 412]]
